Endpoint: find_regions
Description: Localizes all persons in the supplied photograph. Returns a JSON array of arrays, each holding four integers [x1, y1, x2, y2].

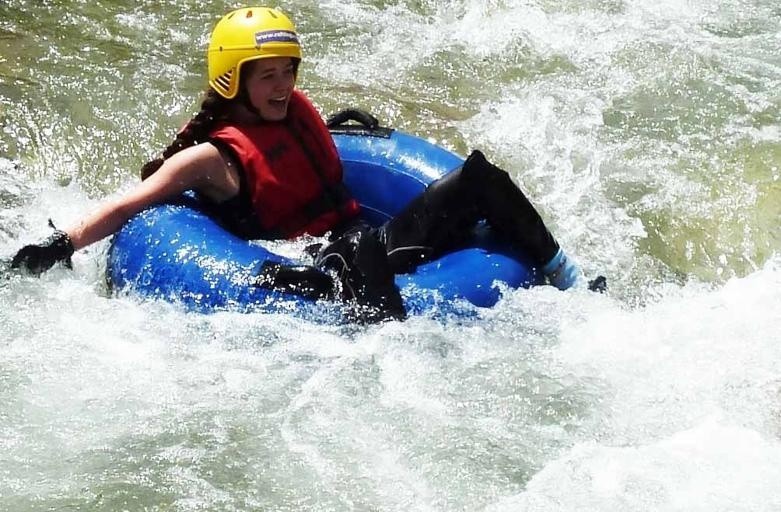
[[11, 6, 606, 323]]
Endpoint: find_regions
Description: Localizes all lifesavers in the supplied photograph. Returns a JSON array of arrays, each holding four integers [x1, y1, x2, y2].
[[106, 107, 546, 324]]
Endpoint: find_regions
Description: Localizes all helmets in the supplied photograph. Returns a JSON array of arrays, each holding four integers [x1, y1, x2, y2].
[[207, 8, 302, 100]]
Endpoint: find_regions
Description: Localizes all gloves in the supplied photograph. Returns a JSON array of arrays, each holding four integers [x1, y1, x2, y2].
[[11, 230, 74, 275]]
